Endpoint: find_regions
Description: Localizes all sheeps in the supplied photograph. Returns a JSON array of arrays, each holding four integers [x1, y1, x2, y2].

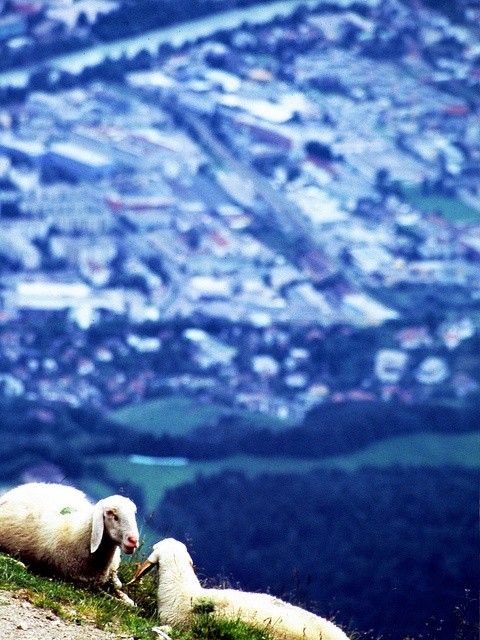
[[127, 537, 351, 639], [0, 482, 136, 605]]
